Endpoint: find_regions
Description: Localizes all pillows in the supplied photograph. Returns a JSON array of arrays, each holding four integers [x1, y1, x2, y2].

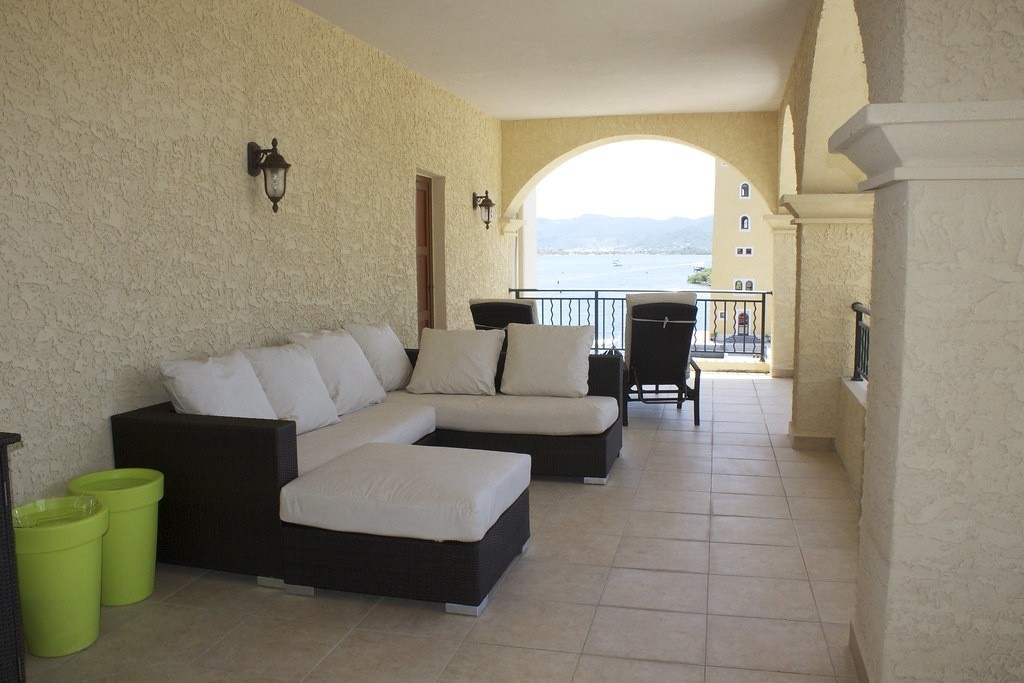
[[157, 352, 280, 421], [286, 328, 385, 415], [404, 328, 504, 396], [343, 323, 415, 393], [238, 349, 340, 430], [499, 324, 592, 396]]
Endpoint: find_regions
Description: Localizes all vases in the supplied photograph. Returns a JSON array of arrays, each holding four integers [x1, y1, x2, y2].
[[15, 497, 110, 659], [66, 467, 164, 613]]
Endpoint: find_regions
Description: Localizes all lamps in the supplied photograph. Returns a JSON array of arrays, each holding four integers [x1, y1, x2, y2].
[[472, 189, 496, 230], [245, 138, 292, 212]]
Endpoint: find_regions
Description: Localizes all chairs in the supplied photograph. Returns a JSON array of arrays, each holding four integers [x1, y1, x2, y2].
[[470, 299, 534, 328], [618, 291, 701, 426]]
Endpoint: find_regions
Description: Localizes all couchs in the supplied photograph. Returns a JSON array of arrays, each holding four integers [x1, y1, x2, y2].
[[110, 346, 626, 618]]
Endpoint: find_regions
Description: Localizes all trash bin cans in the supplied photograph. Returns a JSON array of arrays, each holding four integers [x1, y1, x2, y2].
[[12, 495, 110, 658], [66, 467, 165, 607]]
